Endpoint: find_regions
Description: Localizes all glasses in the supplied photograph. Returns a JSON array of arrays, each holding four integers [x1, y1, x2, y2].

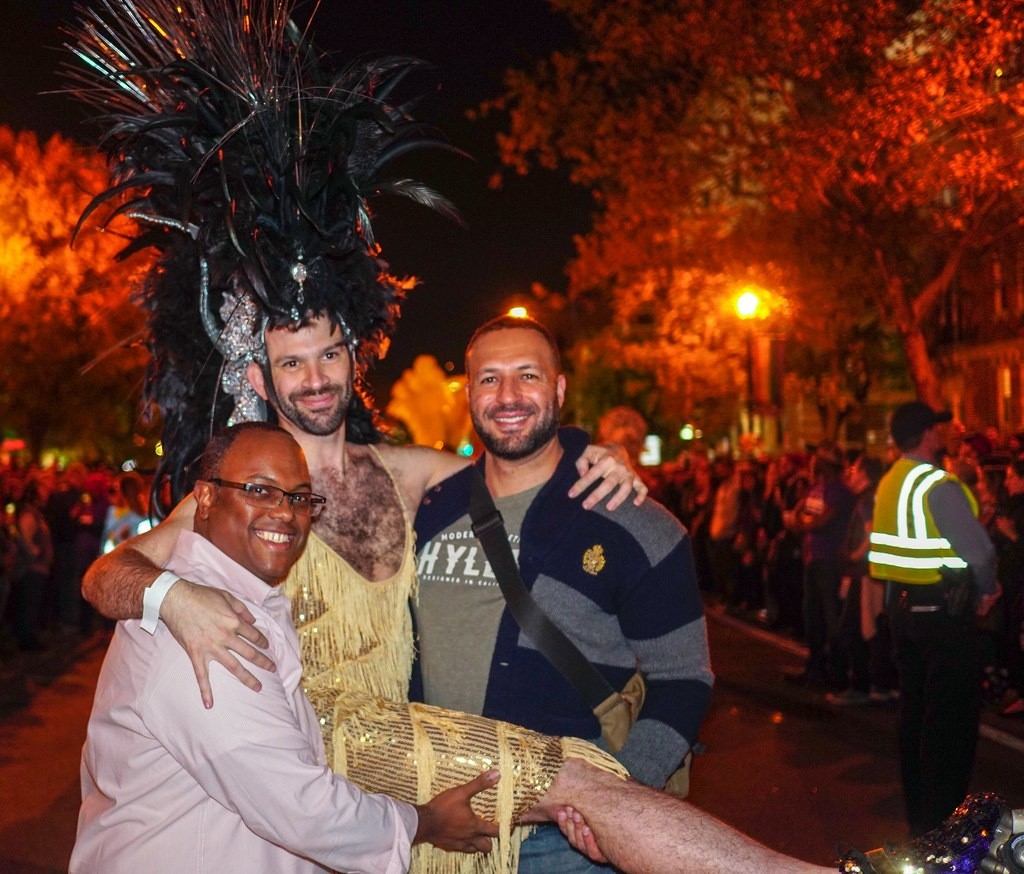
[[210, 479, 327, 519]]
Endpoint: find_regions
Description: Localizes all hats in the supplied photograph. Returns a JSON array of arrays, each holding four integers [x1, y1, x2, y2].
[[886, 400, 953, 452]]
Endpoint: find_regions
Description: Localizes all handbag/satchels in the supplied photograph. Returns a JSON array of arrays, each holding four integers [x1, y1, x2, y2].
[[593, 673, 691, 799]]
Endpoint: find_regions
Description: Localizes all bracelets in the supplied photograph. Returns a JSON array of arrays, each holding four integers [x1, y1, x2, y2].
[[140, 570, 182, 635]]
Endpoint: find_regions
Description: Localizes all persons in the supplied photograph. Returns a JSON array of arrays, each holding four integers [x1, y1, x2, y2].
[[404, 314, 715, 874], [870, 398, 1004, 834], [0, 438, 173, 715], [68, 421, 515, 874], [601, 399, 1024, 704], [80, 293, 1011, 874]]
